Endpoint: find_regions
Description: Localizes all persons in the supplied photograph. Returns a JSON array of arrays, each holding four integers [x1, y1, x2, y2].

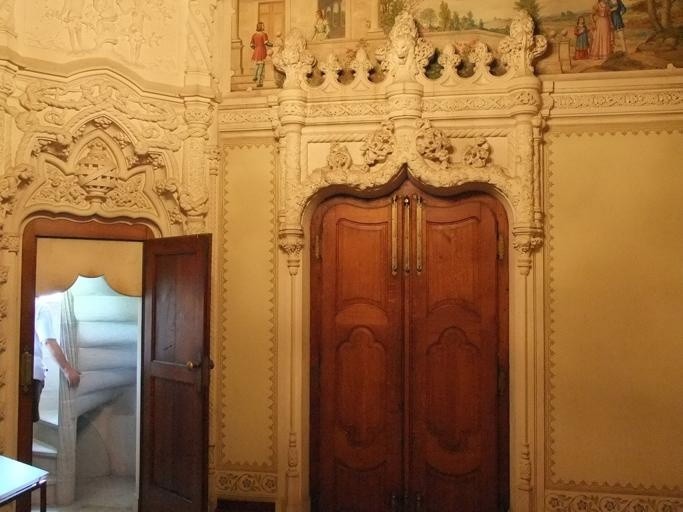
[[249, 21, 273, 87], [607, 0, 628, 54], [32, 295, 83, 424], [588, 0, 613, 61], [572, 15, 590, 61], [310, 8, 331, 41]]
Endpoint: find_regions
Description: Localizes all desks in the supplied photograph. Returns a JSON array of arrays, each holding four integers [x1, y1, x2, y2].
[[0, 454, 51, 512]]
